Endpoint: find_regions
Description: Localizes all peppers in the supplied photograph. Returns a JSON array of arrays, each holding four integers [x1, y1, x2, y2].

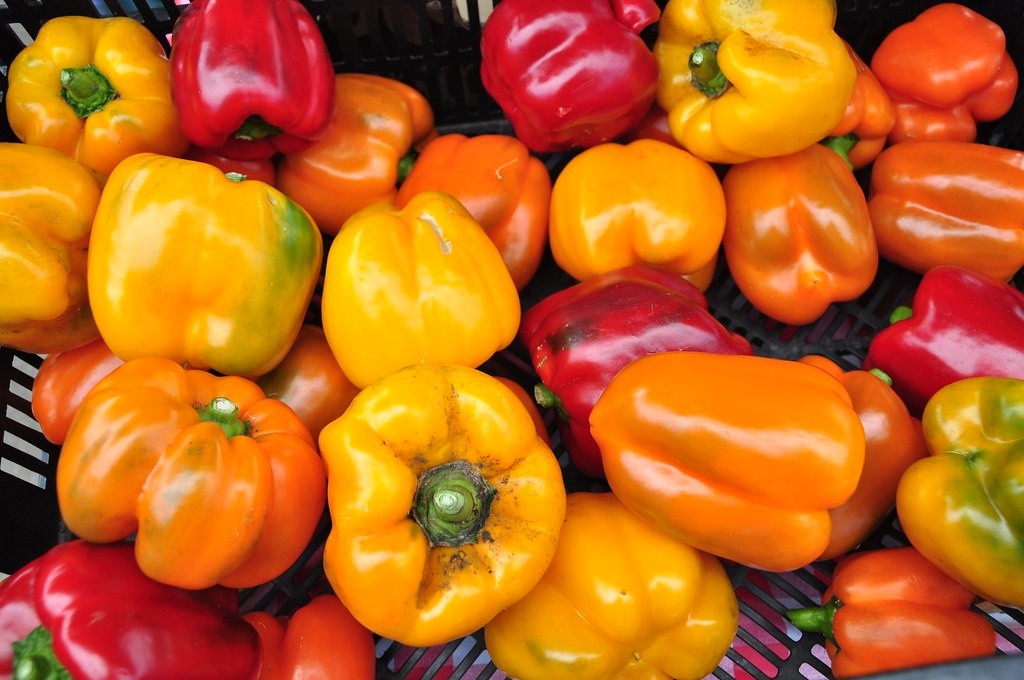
[[0, 1, 1024, 679]]
